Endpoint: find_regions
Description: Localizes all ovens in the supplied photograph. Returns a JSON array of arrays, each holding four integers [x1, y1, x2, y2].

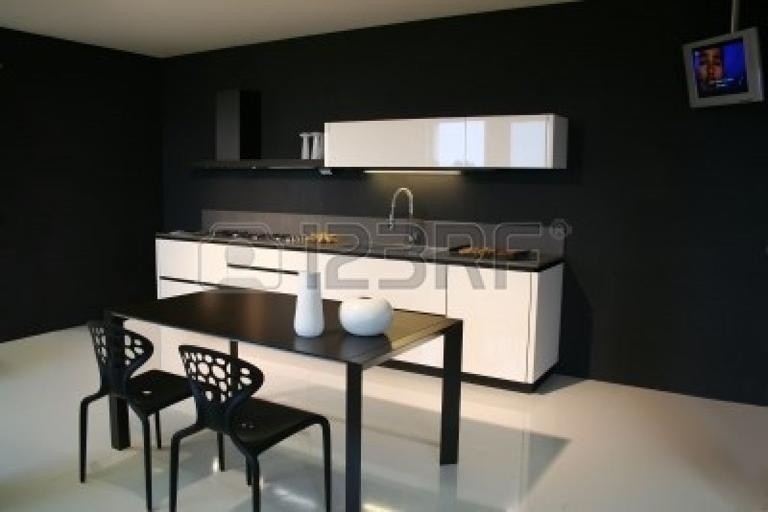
[[198, 246, 310, 295]]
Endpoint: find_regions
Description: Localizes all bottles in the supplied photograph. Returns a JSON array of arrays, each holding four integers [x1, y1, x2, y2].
[[293, 271, 325, 338], [299, 130, 324, 161]]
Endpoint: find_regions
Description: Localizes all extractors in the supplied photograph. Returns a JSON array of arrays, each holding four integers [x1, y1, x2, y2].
[[197, 88, 331, 172]]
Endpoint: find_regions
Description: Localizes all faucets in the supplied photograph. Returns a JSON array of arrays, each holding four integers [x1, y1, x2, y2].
[[387, 186, 415, 244]]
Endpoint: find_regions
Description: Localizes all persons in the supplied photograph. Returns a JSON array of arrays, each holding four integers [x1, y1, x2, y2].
[[693, 47, 722, 95]]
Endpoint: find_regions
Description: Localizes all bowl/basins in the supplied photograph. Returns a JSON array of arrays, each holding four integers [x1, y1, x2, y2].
[[507, 249, 530, 259]]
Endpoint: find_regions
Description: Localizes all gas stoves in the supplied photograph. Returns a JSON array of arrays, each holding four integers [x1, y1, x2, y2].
[[196, 225, 293, 244]]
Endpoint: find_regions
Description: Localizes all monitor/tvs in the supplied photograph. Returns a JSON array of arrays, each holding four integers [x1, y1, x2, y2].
[[681, 26, 765, 107]]
